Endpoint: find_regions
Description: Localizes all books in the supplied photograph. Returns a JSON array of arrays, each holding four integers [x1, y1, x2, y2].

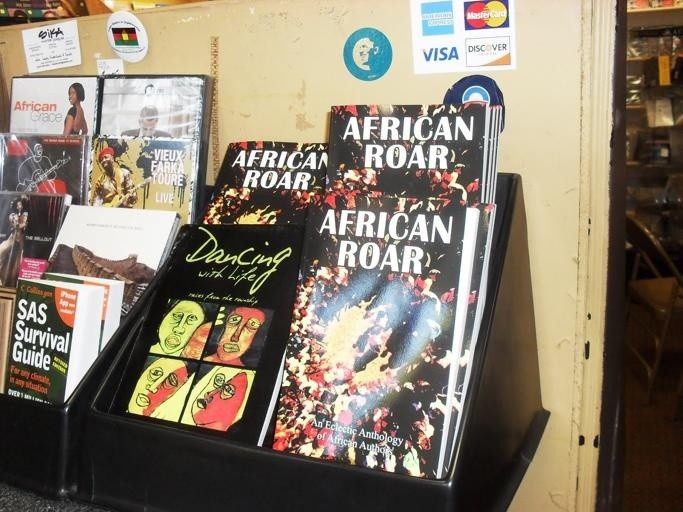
[[126, 104, 502, 483]]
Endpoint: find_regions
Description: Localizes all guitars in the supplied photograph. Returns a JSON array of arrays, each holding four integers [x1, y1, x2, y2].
[[101, 176, 154, 208]]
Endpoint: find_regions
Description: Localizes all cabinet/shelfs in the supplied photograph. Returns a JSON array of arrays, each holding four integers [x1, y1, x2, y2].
[[627, 26, 683, 167]]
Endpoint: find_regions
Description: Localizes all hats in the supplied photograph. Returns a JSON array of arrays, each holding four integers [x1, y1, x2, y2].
[[98, 147, 115, 162]]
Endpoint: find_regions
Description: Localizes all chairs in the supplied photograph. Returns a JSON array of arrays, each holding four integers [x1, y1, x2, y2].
[[624, 211, 683, 402]]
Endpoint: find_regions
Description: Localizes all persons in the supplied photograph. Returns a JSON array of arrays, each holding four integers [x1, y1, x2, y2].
[[61, 83, 87, 137], [0, 196, 29, 288], [89, 147, 137, 208], [121, 106, 171, 139]]
